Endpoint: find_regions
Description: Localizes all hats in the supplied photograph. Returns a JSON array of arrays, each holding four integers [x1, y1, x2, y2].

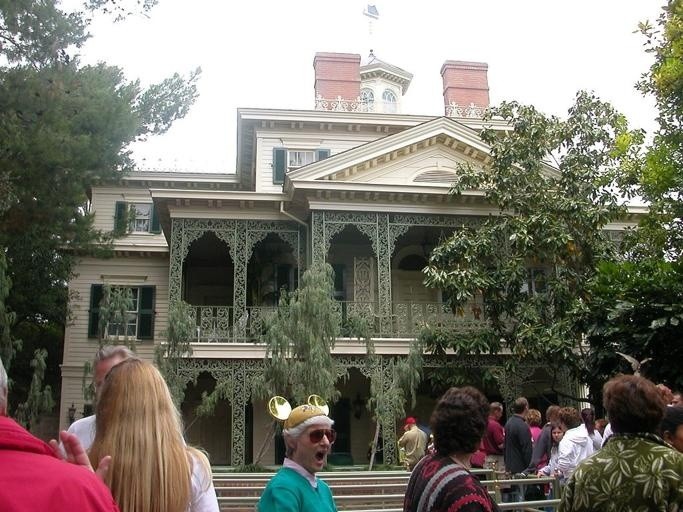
[[266, 394, 329, 429], [405, 417, 415, 424]]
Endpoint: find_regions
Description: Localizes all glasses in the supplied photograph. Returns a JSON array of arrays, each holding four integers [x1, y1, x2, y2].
[[306, 429, 336, 444]]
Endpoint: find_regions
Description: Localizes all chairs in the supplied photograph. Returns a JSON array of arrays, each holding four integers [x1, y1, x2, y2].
[[187, 315, 201, 343], [226, 309, 249, 342], [366, 304, 401, 337]]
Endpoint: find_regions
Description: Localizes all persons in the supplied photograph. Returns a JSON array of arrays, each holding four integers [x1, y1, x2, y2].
[[257, 404, 338, 512], [398, 417, 428, 471], [0, 358, 120, 512], [87, 358, 221, 512], [502, 397, 533, 507], [516, 374, 683, 512], [59, 345, 137, 461], [483, 401, 505, 504], [403, 385, 500, 512]]
